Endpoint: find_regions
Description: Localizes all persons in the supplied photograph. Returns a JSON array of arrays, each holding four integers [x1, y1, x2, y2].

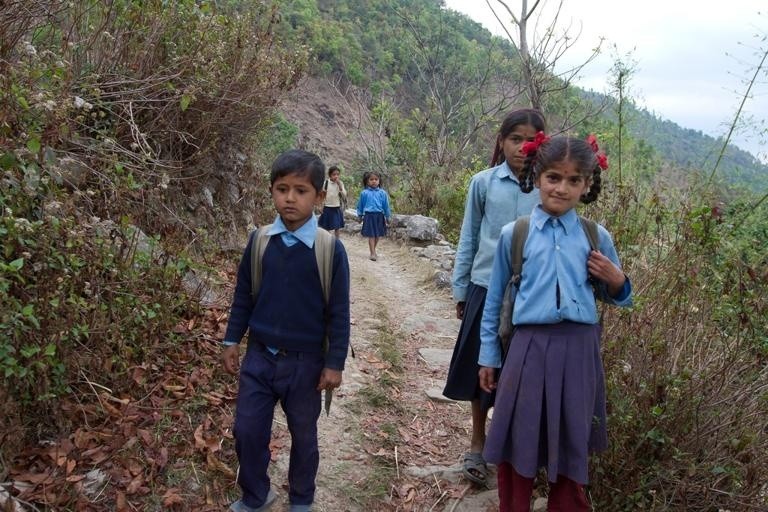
[[356, 170, 392, 261], [473, 134, 635, 512], [317, 166, 348, 241], [221, 149, 353, 512], [443, 109, 550, 494]]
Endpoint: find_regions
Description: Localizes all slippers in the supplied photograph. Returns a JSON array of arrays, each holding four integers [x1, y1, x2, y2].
[[226, 491, 274, 512], [462, 453, 486, 484]]
